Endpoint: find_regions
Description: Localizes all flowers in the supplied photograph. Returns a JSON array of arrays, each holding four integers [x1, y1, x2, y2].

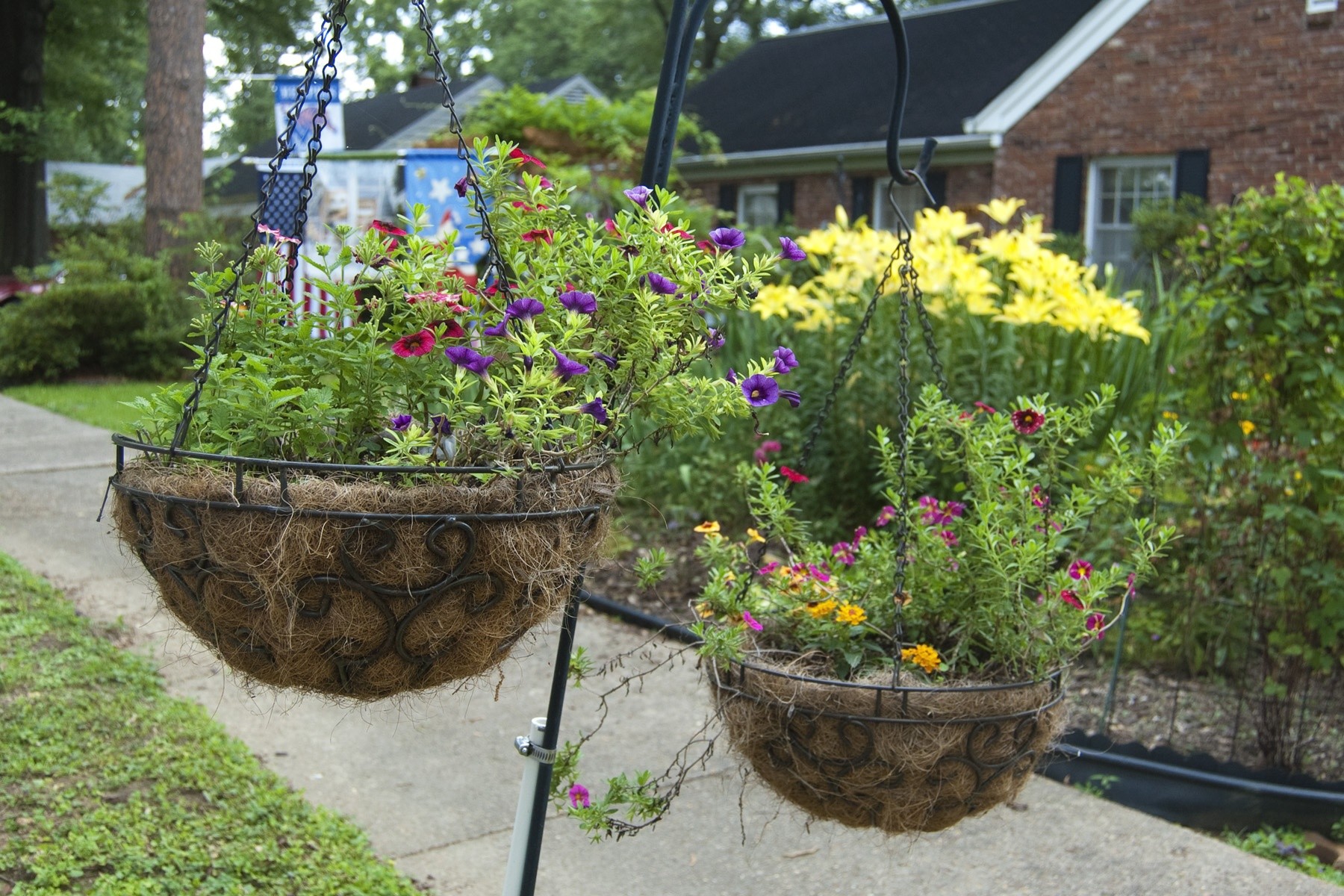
[[547, 382, 1197, 846], [129, 120, 806, 474]]
